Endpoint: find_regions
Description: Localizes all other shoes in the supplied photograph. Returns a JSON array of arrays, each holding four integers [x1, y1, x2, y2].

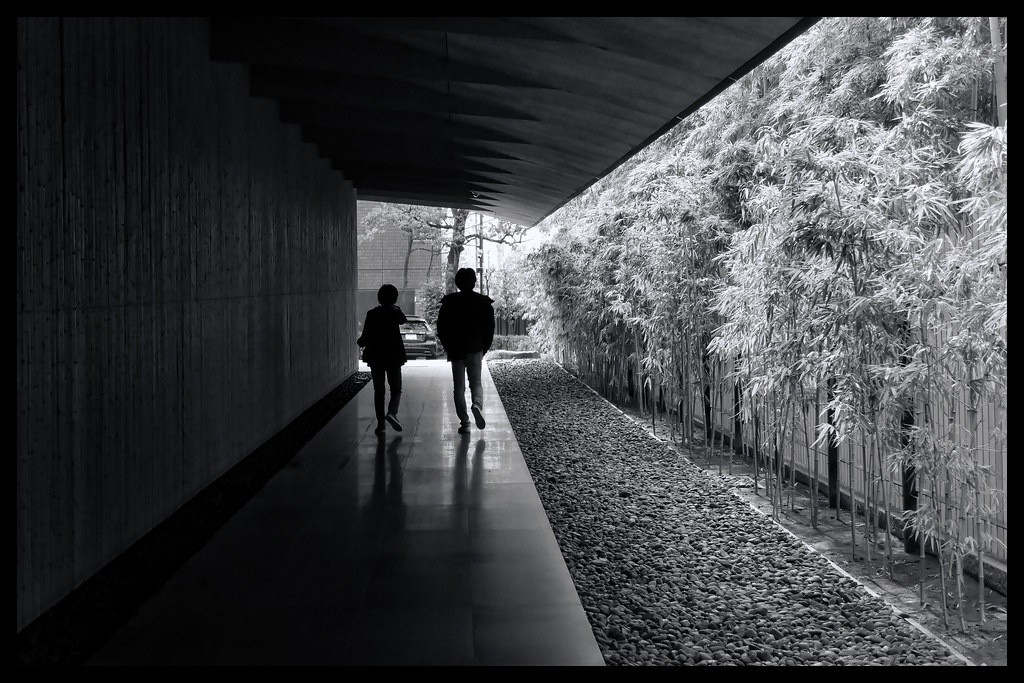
[[374, 427, 387, 439], [471, 405, 486, 430], [458, 426, 471, 434], [385, 412, 403, 432]]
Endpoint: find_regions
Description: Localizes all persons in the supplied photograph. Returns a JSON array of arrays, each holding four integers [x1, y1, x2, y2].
[[436, 268, 495, 433], [357, 284, 407, 433]]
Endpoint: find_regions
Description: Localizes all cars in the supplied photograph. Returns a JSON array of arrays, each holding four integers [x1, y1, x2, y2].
[[399, 315, 437, 360]]
